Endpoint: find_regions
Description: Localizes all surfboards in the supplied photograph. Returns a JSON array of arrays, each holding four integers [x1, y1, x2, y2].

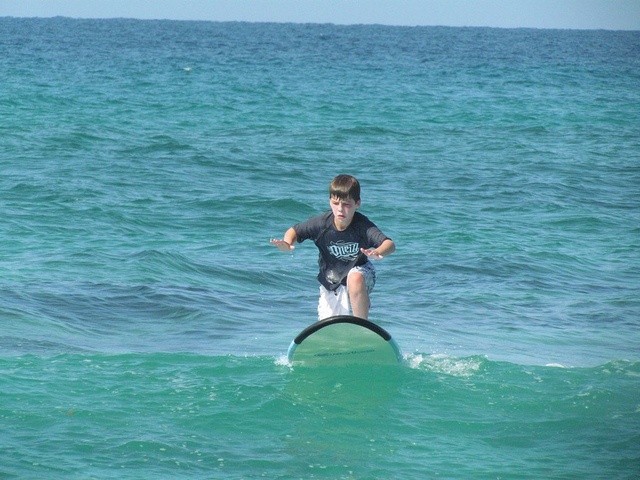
[[287, 314, 406, 368]]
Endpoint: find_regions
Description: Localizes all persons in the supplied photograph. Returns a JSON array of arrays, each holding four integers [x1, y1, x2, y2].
[[270, 174, 396, 320]]
